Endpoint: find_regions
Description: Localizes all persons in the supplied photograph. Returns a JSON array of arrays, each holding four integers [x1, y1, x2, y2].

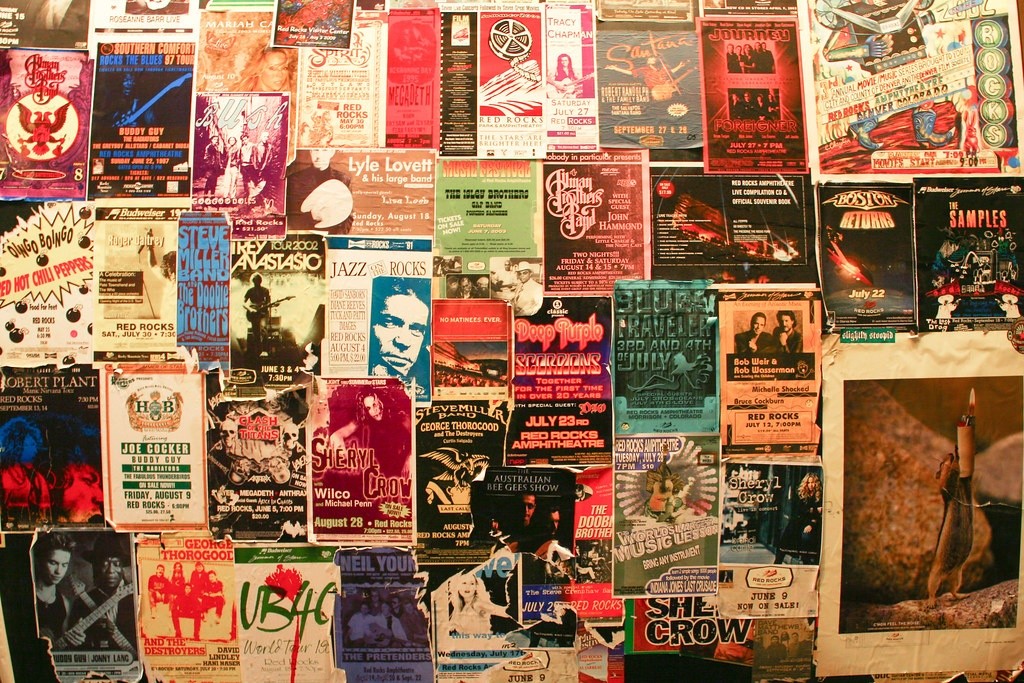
[[148, 562, 225, 639], [448, 574, 511, 634], [200, 129, 272, 198], [735, 311, 803, 354], [731, 88, 778, 120], [229, 44, 290, 93], [328, 389, 411, 481], [32, 532, 133, 645], [755, 626, 812, 657], [495, 492, 560, 560], [511, 261, 543, 315], [773, 473, 822, 564], [446, 273, 491, 298], [283, 148, 353, 233], [370, 290, 429, 383], [726, 42, 774, 72], [242, 275, 272, 341], [344, 591, 428, 648], [546, 53, 584, 98]]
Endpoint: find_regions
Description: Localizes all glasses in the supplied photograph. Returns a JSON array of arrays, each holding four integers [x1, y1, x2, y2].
[[518, 272, 529, 276]]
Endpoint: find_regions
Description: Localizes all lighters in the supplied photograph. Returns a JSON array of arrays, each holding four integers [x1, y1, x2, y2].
[[958, 415, 975, 477]]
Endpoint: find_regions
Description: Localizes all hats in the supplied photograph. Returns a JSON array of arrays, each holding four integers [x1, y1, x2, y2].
[[81, 534, 131, 568], [515, 261, 534, 274]]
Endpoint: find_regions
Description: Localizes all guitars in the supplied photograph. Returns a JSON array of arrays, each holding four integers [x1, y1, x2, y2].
[[40, 582, 134, 652], [246, 295, 296, 323], [113, 73, 193, 128], [68, 571, 137, 658]]
